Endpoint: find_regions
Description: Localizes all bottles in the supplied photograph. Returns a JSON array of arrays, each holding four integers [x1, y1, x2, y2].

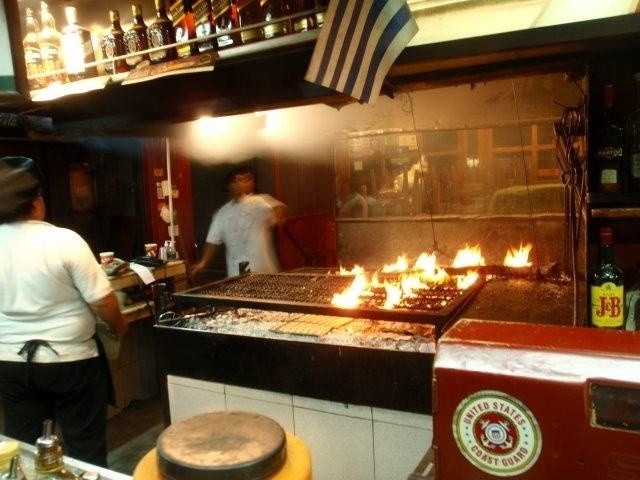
[[147, 0, 175, 63], [213, 0, 236, 48], [290, 0, 316, 32], [588, 227, 626, 331], [22, 6, 42, 88], [169, 0, 191, 61], [63, 4, 100, 80], [590, 83, 633, 207], [103, 8, 127, 75], [316, 0, 328, 29], [238, 0, 262, 41], [126, 3, 144, 65], [193, 0, 212, 51], [37, 1, 64, 86], [263, 0, 292, 38], [37, 418, 61, 475]]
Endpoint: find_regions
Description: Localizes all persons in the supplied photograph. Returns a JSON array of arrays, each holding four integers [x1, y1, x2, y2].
[[0, 157, 128, 469], [358, 183, 378, 218], [190, 167, 289, 275], [337, 177, 366, 220]]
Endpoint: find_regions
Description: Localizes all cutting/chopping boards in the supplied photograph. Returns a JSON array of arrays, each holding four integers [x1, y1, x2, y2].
[[155, 413, 288, 480]]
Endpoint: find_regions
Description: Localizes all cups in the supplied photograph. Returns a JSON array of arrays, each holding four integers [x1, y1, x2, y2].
[[145, 244, 157, 259], [100, 253, 113, 271]]
